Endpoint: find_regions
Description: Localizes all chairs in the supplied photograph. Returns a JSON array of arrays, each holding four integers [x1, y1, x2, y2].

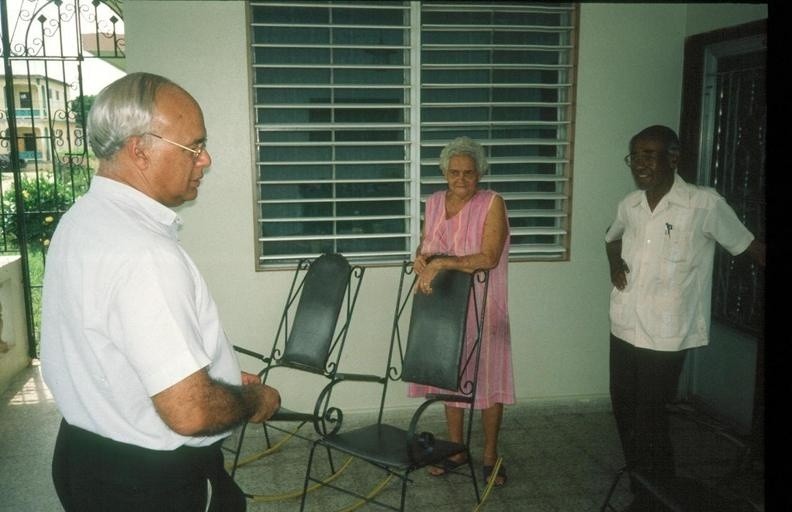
[[598, 404, 765, 512], [222, 251, 502, 511]]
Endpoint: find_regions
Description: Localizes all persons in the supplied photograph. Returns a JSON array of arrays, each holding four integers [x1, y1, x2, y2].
[[604, 123, 765, 512], [38, 71, 282, 512], [412, 135, 519, 488]]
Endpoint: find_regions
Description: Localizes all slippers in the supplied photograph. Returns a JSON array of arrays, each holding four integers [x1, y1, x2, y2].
[[428, 459, 467, 477], [484, 463, 506, 487]]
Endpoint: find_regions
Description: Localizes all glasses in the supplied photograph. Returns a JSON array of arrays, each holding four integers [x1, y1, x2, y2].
[[149, 133, 204, 158]]
